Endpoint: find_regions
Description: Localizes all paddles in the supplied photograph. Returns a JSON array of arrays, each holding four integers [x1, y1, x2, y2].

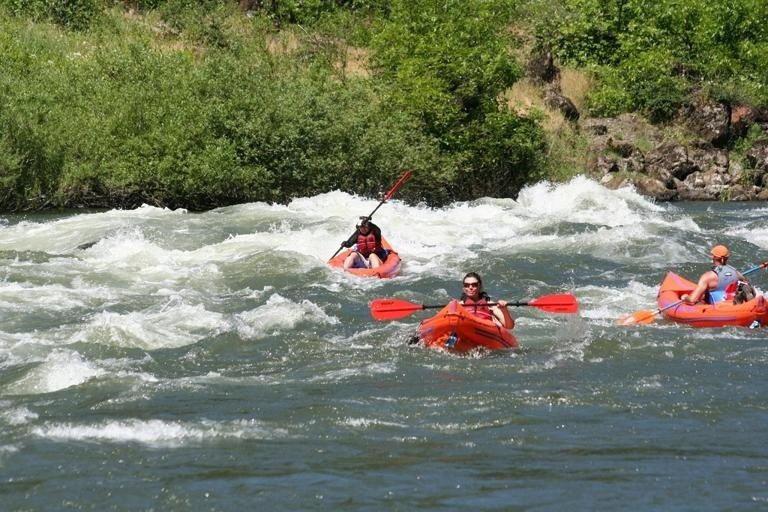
[[371, 294, 578, 320], [329, 169, 412, 260], [616, 262, 767, 324]]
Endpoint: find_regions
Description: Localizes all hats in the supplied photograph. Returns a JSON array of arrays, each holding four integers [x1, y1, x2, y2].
[[711, 246, 728, 259]]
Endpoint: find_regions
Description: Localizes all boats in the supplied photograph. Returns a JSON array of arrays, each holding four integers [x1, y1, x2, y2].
[[330, 234, 401, 279], [416, 299, 516, 352], [659, 268, 768, 327]]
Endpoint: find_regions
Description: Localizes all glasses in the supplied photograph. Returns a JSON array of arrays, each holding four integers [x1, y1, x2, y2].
[[463, 283, 478, 287]]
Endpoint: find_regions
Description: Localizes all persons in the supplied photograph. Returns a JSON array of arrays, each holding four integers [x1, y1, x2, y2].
[[449, 272, 515, 330], [680, 245, 745, 306], [341, 215, 388, 270]]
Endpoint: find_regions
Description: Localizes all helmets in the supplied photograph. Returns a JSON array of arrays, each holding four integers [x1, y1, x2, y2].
[[358, 219, 368, 227]]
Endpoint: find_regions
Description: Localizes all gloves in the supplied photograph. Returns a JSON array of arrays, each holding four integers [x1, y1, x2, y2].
[[362, 220, 368, 226], [341, 241, 348, 246]]
